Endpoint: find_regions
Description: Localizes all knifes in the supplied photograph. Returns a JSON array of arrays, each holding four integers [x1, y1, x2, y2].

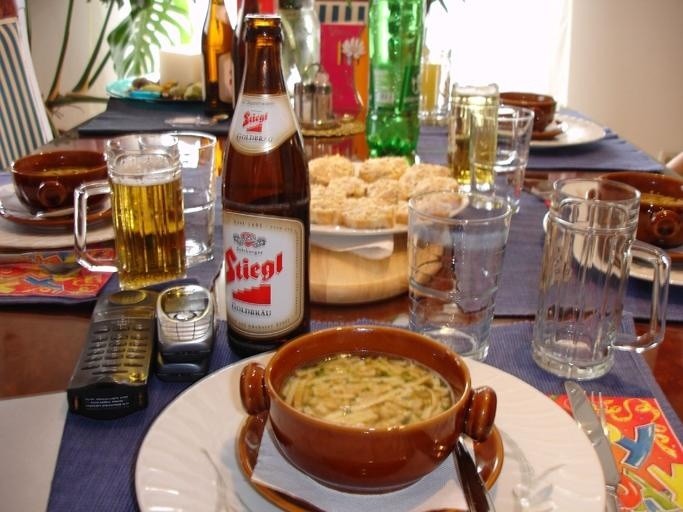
[[565, 377, 624, 511]]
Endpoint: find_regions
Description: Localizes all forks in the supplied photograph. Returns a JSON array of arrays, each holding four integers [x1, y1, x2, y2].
[[584, 389, 610, 441]]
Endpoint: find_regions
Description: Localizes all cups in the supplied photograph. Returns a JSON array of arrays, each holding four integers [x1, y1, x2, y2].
[[408, 191, 512, 364], [73, 152, 189, 290], [471, 106, 535, 218], [418, 48, 454, 126], [293, 81, 306, 123], [172, 133, 215, 266], [446, 83, 499, 183], [302, 79, 314, 125], [315, 84, 331, 124], [531, 178, 669, 382]]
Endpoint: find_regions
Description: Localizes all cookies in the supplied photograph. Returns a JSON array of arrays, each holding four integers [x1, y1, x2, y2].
[[308, 155, 463, 229]]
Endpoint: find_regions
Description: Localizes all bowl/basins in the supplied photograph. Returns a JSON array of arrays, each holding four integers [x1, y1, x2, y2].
[[12, 150, 111, 212], [599, 173, 682, 249], [241, 328, 498, 495], [499, 92, 558, 130]]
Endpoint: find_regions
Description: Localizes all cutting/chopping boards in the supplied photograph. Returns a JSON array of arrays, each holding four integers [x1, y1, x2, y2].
[[304, 242, 456, 305]]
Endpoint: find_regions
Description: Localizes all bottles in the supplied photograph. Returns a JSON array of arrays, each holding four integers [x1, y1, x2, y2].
[[367, 1, 426, 157], [201, 0, 238, 118], [223, 14, 309, 357], [292, 81, 312, 121], [233, 0, 259, 105], [314, 73, 333, 119], [277, 0, 321, 108]]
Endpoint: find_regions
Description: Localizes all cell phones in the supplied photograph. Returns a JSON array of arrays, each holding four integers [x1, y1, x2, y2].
[[156, 349, 209, 383], [156, 285, 215, 361], [65, 290, 157, 420]]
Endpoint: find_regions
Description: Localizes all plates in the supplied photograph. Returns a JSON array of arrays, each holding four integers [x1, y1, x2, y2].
[[135, 341, 610, 512], [524, 114, 606, 150], [0, 191, 120, 229], [542, 211, 682, 285], [236, 409, 506, 512], [305, 190, 471, 236], [0, 183, 148, 249], [497, 119, 569, 139]]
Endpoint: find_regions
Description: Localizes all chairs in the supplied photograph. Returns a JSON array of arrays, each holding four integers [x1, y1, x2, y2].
[[0, 0, 54, 175]]
